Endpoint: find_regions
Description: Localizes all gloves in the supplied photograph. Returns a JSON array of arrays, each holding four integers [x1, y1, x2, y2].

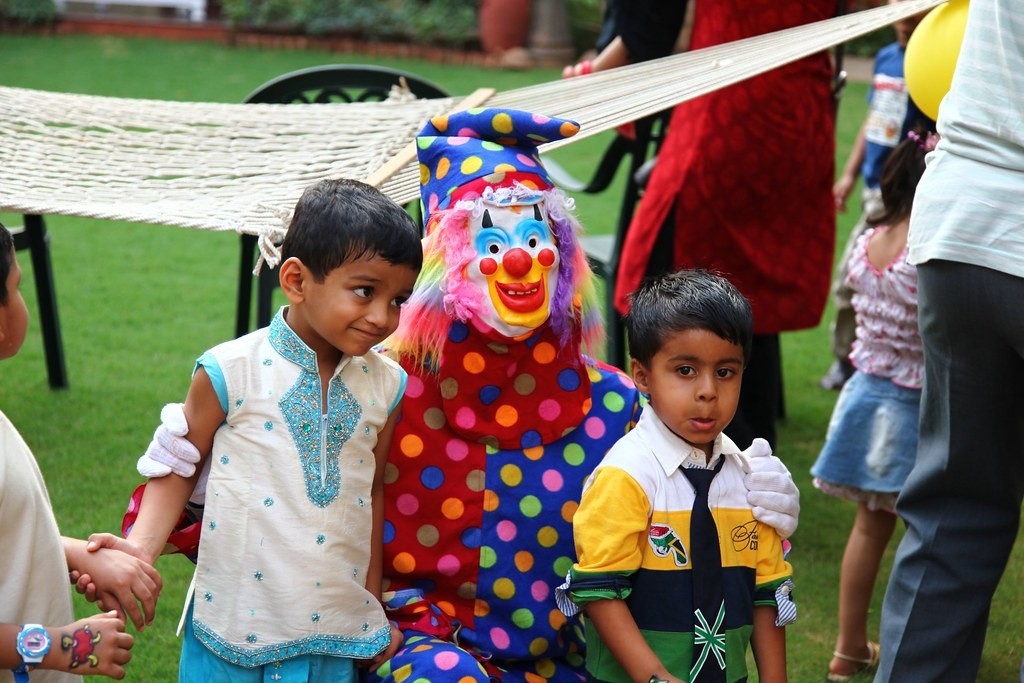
[[741, 438, 800, 541], [137, 403, 213, 506]]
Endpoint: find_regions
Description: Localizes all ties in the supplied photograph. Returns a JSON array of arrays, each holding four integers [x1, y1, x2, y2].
[[678, 453, 726, 683]]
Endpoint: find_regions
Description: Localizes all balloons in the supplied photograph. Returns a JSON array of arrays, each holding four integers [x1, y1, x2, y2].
[[904, 0, 970, 121]]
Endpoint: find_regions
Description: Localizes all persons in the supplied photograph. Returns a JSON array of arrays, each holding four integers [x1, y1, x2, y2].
[[0, 222, 163, 683], [69, 177, 423, 683], [615, 0, 838, 455], [556, 268, 797, 683], [123, 110, 801, 683], [564, 0, 687, 78], [808, 0, 1024, 683]]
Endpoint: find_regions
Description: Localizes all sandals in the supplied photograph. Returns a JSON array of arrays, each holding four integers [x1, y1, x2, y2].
[[827, 641, 880, 682]]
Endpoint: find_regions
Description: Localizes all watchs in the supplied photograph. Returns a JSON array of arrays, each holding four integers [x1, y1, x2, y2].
[[12, 624, 50, 683]]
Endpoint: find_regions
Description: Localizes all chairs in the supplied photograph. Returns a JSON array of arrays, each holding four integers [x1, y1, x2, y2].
[[579, 107, 672, 371], [235, 63, 453, 339], [9, 214, 67, 390]]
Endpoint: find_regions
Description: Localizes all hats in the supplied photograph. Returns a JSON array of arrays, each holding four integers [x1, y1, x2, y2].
[[415, 107, 580, 238]]
